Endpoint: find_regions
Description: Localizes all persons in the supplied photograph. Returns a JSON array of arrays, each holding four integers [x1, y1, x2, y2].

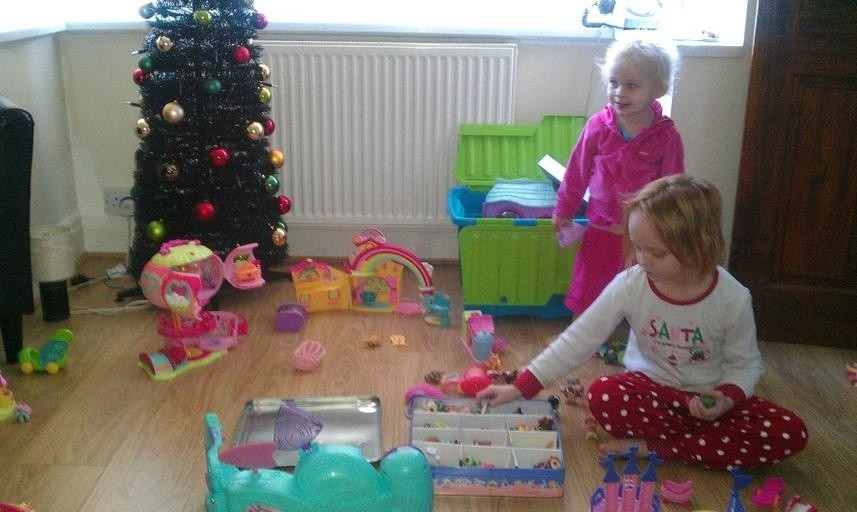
[[476, 171, 808, 474], [553, 37, 683, 324]]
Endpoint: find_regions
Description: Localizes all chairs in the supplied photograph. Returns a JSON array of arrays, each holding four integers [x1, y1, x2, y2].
[[0, 97, 34, 364]]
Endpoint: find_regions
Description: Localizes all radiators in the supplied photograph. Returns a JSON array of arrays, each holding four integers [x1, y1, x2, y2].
[[249, 40, 518, 231]]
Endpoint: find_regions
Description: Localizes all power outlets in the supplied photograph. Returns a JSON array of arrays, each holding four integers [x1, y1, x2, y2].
[[103, 187, 134, 218]]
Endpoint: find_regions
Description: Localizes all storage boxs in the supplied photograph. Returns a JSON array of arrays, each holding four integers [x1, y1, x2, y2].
[[446, 115, 592, 321]]
